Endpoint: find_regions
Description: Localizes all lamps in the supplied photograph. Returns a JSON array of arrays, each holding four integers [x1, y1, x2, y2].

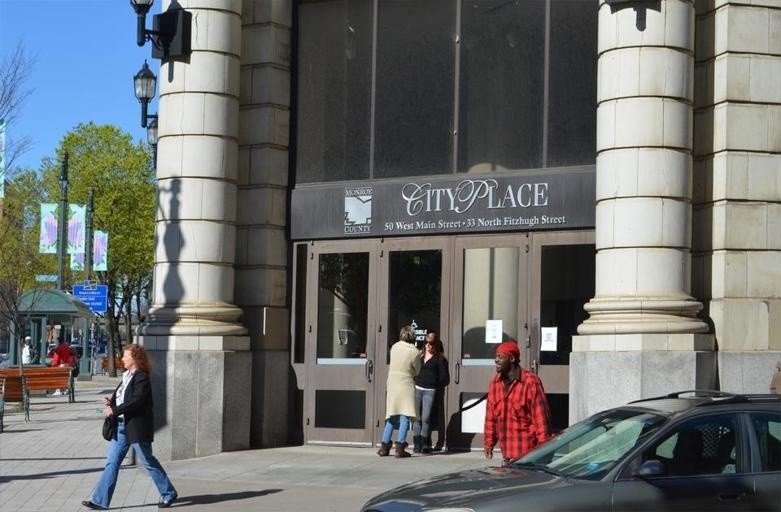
[[127, 1, 193, 64], [133, 57, 158, 127], [148, 112, 158, 171]]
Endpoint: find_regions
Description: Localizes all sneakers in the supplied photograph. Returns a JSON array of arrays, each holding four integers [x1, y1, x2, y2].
[[82, 500, 108, 510], [53, 389, 70, 396], [159, 494, 178, 507]]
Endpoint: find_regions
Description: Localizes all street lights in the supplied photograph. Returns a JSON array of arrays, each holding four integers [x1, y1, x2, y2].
[[48, 151, 69, 395], [77, 187, 96, 382]]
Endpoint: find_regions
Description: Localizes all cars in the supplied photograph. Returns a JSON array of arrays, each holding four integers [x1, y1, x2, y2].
[[35, 340, 109, 370], [360, 390, 781, 511]]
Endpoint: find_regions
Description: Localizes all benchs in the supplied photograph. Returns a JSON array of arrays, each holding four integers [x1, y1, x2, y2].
[[0, 362, 76, 434], [102, 357, 126, 375]]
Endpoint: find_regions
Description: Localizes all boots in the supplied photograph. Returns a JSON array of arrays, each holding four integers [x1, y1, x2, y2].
[[377, 440, 394, 456], [395, 441, 413, 457], [413, 435, 421, 453], [421, 436, 433, 454]]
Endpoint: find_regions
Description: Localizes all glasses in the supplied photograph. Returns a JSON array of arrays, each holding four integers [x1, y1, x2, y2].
[[425, 340, 434, 345]]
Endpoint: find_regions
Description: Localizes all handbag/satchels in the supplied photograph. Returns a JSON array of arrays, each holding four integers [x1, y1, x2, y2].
[[71, 366, 80, 377], [101, 418, 113, 441]]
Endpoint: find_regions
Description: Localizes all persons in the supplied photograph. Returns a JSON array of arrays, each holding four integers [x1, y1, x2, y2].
[[412, 333, 450, 454], [82, 345, 177, 511], [483, 341, 553, 464], [21, 336, 40, 365], [377, 326, 421, 458], [51, 336, 76, 397]]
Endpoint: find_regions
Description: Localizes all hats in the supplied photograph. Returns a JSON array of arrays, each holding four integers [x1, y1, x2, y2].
[[496, 341, 521, 357], [25, 336, 32, 340]]
